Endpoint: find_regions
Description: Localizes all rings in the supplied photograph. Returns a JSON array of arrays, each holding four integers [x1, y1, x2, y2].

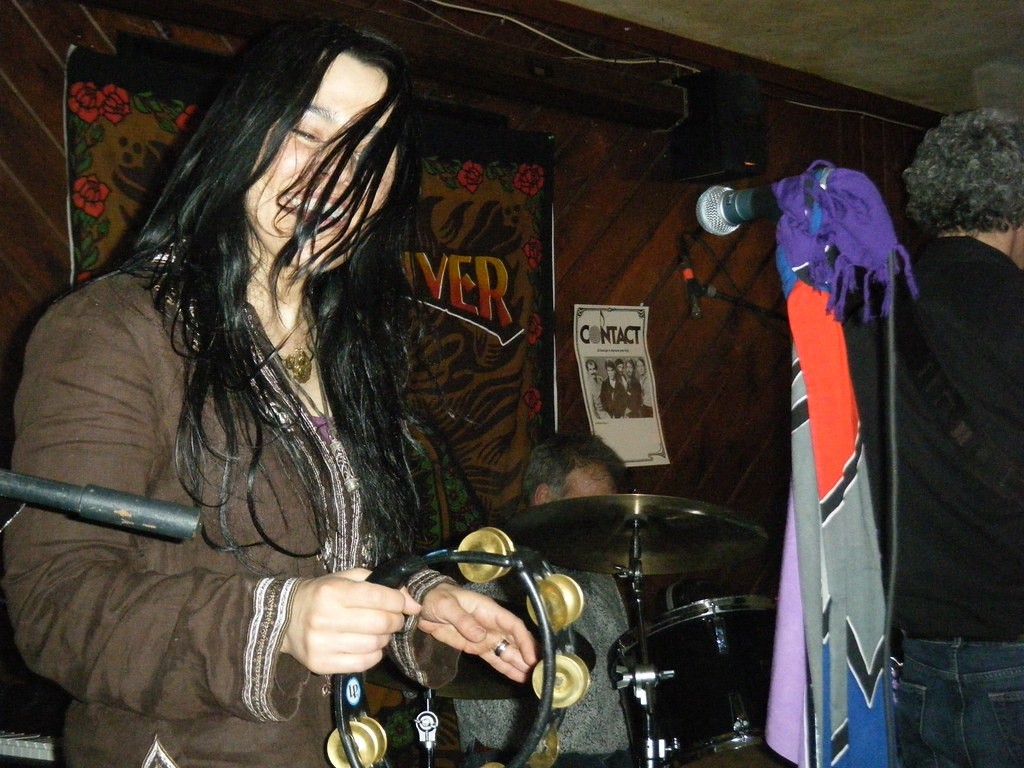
[[495, 639, 510, 656]]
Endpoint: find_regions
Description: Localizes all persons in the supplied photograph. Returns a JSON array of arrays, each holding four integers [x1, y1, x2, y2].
[[3, 17, 542, 768], [585, 358, 655, 421], [869, 109, 1024, 768], [522, 431, 634, 508]]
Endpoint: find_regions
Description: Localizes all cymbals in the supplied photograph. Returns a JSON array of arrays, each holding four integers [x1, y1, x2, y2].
[[499, 494, 771, 578], [322, 524, 590, 768]]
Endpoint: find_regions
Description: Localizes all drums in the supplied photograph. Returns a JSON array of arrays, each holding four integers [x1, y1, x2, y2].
[[602, 593, 799, 768]]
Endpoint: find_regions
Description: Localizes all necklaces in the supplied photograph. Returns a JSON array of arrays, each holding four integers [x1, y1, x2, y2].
[[255, 298, 316, 386]]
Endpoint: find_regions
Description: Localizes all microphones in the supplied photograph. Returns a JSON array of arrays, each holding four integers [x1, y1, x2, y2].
[[694, 169, 829, 237], [677, 238, 704, 321]]
